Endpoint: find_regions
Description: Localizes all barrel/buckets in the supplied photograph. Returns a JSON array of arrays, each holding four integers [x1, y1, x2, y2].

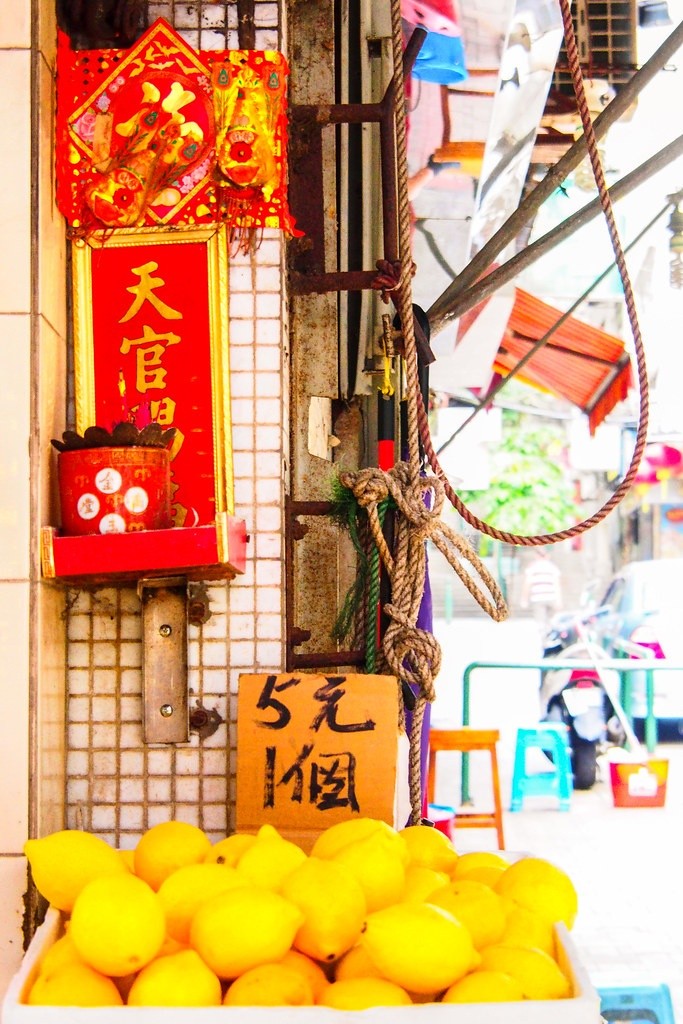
[[605, 761, 669, 807]]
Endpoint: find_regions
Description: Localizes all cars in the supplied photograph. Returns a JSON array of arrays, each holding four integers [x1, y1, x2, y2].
[[596, 559, 683, 739]]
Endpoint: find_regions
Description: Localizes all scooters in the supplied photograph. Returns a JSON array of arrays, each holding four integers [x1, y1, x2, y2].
[[537, 602, 616, 793]]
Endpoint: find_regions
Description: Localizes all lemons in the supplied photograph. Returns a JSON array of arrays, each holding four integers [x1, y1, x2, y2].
[[23, 817, 577, 1009]]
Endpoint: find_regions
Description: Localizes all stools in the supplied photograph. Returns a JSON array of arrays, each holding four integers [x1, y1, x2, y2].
[[511, 720, 574, 811], [429, 727, 506, 851]]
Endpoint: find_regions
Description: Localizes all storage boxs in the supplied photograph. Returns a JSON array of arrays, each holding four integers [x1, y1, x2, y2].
[[0, 848, 600, 1024]]
